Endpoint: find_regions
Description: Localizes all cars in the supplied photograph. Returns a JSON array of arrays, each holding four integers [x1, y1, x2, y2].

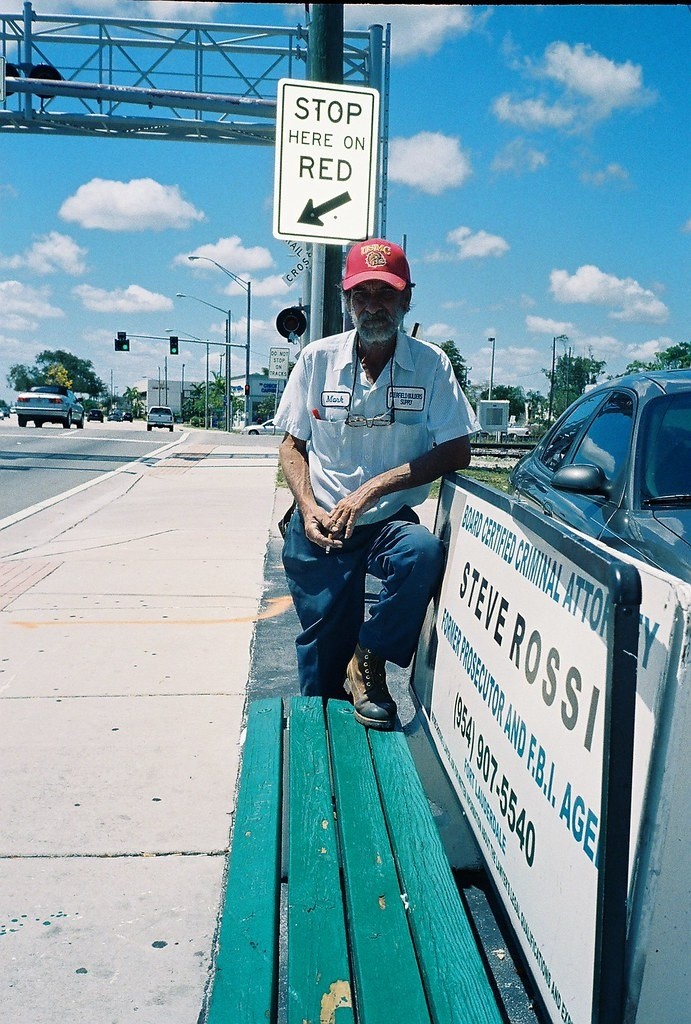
[[242, 418, 286, 435], [107, 409, 122, 422], [87, 409, 104, 423], [506, 366, 691, 588], [145, 406, 175, 432], [0, 406, 16, 420], [121, 411, 133, 422], [15, 385, 85, 429]]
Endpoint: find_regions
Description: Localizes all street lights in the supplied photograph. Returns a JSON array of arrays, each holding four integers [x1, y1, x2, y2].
[[488, 337, 495, 401], [176, 292, 231, 433], [143, 375, 160, 406], [188, 256, 251, 427], [548, 335, 566, 430], [165, 328, 208, 431]]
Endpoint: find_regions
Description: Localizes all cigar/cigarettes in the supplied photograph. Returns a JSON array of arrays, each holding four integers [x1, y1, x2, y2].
[[325, 533, 332, 553]]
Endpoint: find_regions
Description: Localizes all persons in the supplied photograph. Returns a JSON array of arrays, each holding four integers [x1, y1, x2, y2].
[[273, 239, 483, 730], [257, 417, 262, 425]]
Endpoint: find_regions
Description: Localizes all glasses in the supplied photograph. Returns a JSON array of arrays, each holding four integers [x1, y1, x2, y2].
[[344, 395, 395, 427]]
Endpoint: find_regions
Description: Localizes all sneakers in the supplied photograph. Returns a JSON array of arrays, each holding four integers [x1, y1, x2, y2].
[[343, 643, 397, 729]]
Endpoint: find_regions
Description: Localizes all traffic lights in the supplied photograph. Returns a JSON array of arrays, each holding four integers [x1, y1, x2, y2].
[[170, 336, 178, 355], [245, 384, 250, 395], [115, 331, 130, 351]]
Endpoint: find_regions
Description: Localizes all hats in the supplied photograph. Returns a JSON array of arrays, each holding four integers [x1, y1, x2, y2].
[[342, 238, 411, 292]]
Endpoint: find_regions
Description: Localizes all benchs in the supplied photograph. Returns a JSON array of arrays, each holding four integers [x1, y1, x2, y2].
[[205, 472, 691, 1024]]
